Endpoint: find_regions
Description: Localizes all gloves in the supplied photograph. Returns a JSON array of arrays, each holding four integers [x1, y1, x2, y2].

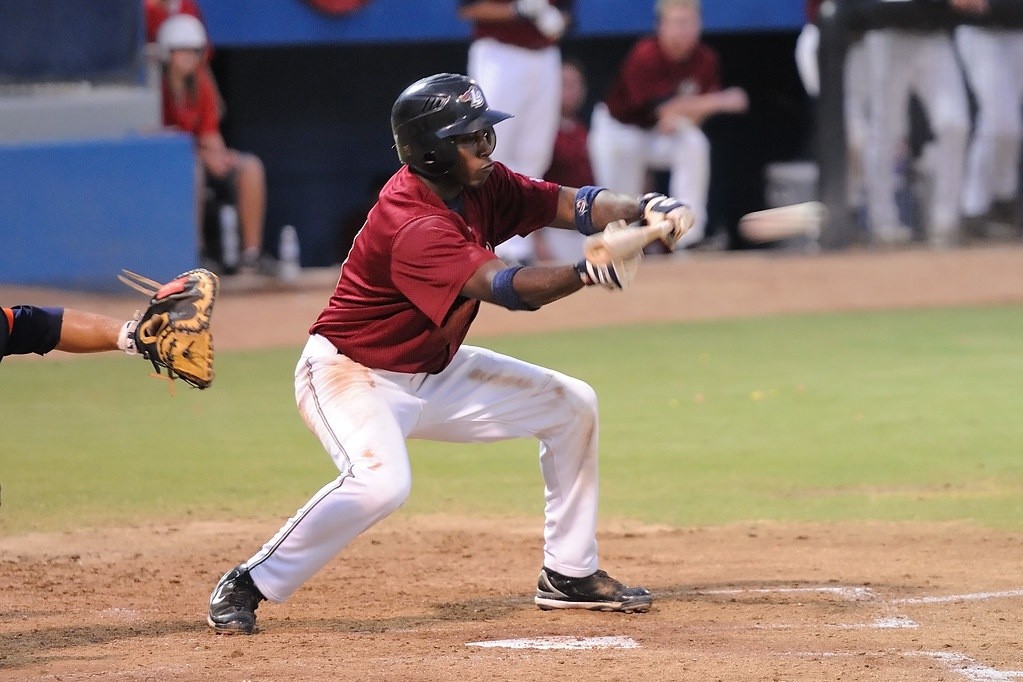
[[637, 191, 695, 252], [577, 218, 674, 292]]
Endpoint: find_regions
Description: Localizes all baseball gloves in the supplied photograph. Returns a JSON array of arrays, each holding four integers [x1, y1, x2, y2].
[[117, 267, 220, 391]]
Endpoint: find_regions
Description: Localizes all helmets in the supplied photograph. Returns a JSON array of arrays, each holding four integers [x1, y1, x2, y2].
[[156, 12, 208, 63], [390, 73, 516, 177]]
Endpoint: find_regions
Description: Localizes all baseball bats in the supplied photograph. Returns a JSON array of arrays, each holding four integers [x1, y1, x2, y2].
[[582, 219, 676, 267]]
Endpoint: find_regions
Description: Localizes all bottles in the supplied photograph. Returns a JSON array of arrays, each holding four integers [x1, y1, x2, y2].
[[279, 224, 300, 289], [217, 204, 239, 268]]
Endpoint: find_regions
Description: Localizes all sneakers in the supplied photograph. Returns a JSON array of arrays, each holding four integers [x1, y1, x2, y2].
[[534, 565, 653, 615], [206, 561, 267, 635]]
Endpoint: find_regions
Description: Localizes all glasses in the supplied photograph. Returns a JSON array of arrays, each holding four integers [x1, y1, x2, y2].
[[451, 128, 489, 146]]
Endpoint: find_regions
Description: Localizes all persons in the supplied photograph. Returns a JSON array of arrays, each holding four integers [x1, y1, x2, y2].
[[207, 73, 695, 635], [457, 0, 594, 263], [586, 0, 749, 253], [739, 0, 1023, 249], [147, 0, 266, 277], [0, 267, 220, 399]]
